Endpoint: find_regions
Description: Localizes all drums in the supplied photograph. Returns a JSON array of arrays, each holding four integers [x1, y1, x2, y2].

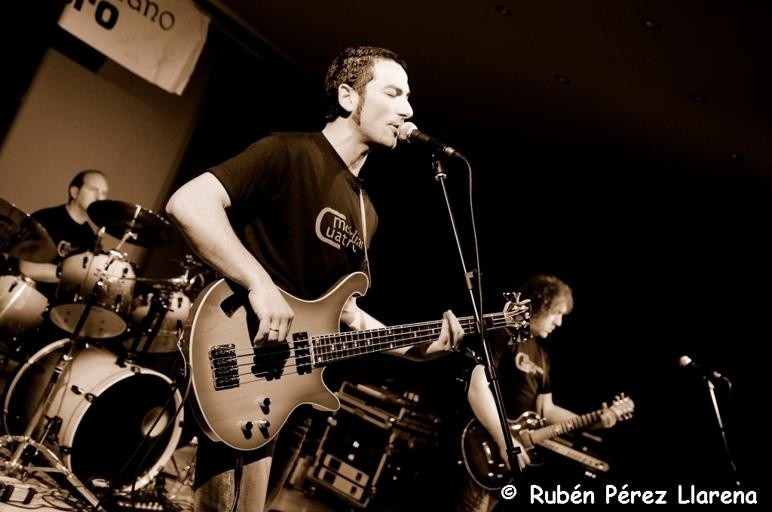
[[0, 273, 51, 362], [49, 247, 136, 339], [122, 289, 191, 354], [3, 338, 186, 497]]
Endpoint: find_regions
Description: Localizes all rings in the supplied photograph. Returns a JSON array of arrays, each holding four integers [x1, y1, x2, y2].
[[269, 328, 280, 332]]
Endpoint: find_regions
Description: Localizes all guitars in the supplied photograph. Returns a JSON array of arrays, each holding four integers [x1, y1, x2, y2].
[[460, 392, 635, 490], [182, 271, 533, 453]]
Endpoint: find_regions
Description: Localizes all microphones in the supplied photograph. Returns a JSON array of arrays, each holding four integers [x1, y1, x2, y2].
[[679, 356, 728, 382], [396, 121, 461, 158]]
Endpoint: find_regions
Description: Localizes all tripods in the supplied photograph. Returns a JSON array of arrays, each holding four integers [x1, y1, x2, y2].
[[0, 237, 106, 512]]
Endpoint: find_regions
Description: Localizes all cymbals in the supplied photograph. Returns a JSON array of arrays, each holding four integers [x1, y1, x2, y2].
[[87, 198, 176, 248], [0, 197, 57, 263], [159, 258, 212, 278]]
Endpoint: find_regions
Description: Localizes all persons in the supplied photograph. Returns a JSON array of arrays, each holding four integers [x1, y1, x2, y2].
[[7, 168, 109, 308], [457, 273, 617, 511], [163, 42, 464, 512]]
